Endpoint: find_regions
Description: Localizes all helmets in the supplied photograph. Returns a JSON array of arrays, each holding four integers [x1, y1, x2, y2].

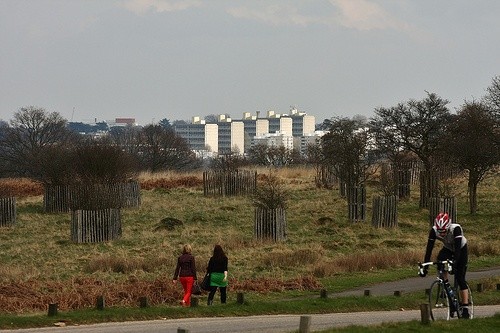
[[435, 212, 451, 230]]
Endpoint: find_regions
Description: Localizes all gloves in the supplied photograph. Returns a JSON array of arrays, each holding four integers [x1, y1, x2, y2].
[[448, 262, 457, 275], [419, 266, 428, 277]]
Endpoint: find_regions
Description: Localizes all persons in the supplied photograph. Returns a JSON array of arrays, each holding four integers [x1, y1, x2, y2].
[[172, 244, 198, 308], [206, 244, 229, 305], [419, 213, 470, 318]]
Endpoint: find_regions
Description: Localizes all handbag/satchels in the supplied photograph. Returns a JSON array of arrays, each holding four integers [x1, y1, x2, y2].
[[201, 271, 210, 291]]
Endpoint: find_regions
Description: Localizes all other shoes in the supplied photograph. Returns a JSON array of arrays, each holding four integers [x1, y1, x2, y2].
[[463, 306, 469, 319], [440, 290, 453, 298], [180, 300, 187, 307]]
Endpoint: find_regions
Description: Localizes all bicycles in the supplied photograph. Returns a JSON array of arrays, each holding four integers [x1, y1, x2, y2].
[[416, 259, 474, 322]]
